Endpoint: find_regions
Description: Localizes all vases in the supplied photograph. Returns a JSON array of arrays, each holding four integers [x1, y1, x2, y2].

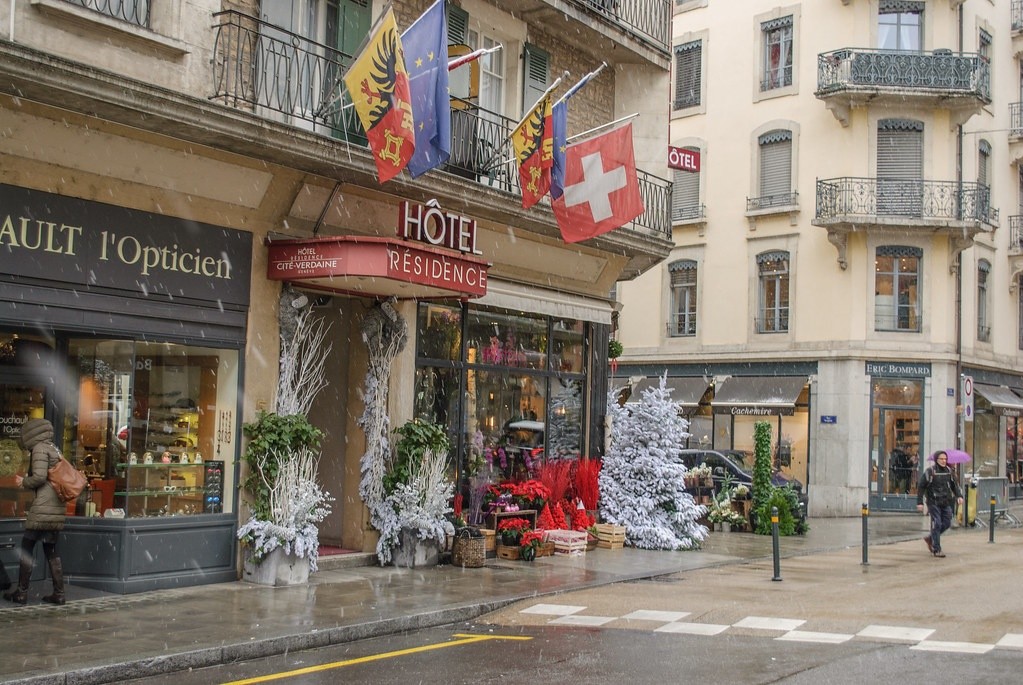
[[387, 527, 439, 568], [542, 542, 555, 555], [497, 545, 521, 560], [685, 475, 714, 503], [714, 523, 722, 531], [502, 533, 516, 546], [722, 521, 731, 532], [516, 534, 523, 544], [522, 547, 536, 561], [242, 540, 310, 586]]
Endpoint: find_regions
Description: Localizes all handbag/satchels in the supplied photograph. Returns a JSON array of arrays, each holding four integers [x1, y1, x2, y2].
[[46, 444, 88, 503]]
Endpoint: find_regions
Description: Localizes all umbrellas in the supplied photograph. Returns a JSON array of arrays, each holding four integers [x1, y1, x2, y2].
[[927, 449, 971, 462]]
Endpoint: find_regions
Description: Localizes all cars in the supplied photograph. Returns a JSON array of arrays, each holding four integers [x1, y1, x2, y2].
[[678, 448, 811, 520]]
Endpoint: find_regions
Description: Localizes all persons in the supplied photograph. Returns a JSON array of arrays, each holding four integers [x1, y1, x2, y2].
[[773, 439, 792, 470], [493, 409, 543, 477], [3, 418, 67, 605], [699, 435, 711, 449], [916, 451, 963, 557], [888, 443, 916, 494]]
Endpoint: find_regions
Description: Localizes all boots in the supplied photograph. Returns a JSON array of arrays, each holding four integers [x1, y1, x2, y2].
[[42, 556, 65, 604], [4, 558, 33, 604]]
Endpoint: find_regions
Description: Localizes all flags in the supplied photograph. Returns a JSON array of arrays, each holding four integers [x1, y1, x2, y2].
[[343, 7, 415, 184], [550, 123, 647, 245], [550, 75, 590, 201], [512, 85, 559, 208], [400, 0, 451, 180]]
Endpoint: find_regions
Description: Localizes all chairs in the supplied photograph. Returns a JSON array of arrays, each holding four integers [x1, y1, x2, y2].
[[477, 139, 511, 192]]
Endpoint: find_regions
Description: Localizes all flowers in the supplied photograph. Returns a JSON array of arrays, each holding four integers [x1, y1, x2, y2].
[[535, 455, 604, 529], [235, 411, 336, 575], [370, 417, 456, 567], [707, 491, 747, 527], [481, 479, 551, 512], [497, 517, 543, 550]]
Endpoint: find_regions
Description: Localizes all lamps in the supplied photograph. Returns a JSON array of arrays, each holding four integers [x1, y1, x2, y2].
[[286, 282, 309, 308]]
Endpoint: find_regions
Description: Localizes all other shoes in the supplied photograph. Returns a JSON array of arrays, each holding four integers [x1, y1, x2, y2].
[[934, 550, 945, 557], [925, 536, 933, 552]]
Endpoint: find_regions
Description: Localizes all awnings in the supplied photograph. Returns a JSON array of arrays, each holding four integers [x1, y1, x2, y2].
[[624, 377, 714, 415], [973, 384, 1023, 416], [711, 377, 808, 416]]
